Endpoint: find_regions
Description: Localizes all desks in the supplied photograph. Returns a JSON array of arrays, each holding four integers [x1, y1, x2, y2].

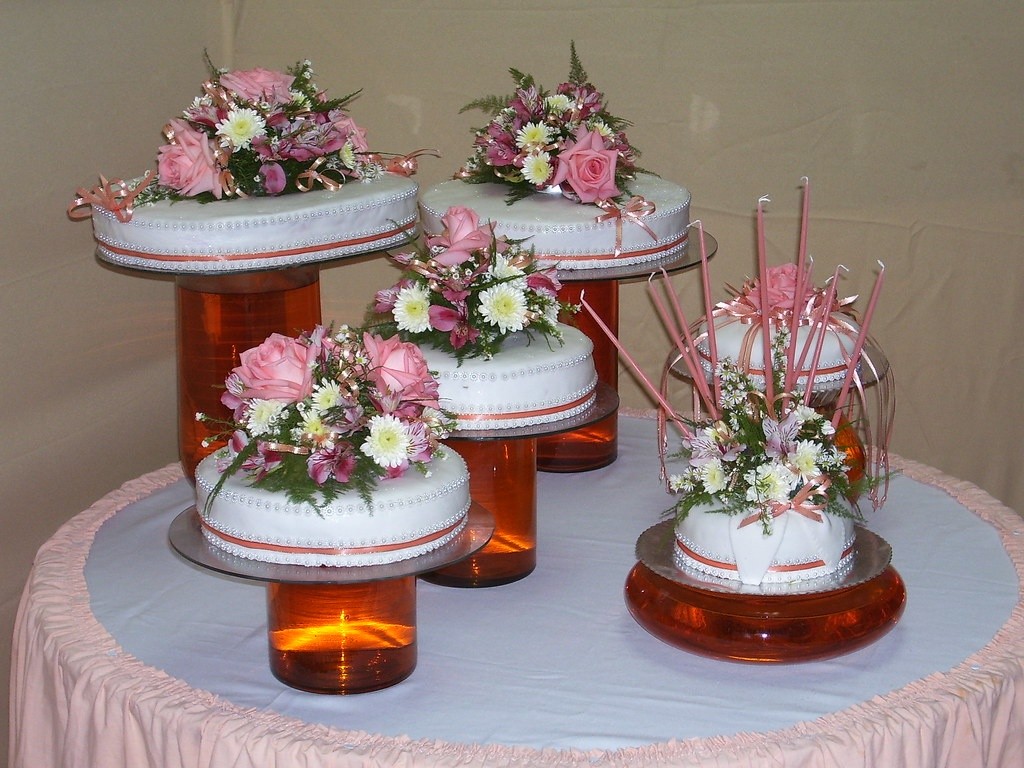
[[13, 416, 1024, 768]]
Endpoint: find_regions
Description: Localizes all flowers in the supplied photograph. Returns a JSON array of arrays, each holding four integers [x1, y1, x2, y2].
[[454, 36, 632, 208], [374, 207, 567, 358], [733, 261, 822, 326], [668, 334, 854, 531], [159, 60, 362, 206], [223, 326, 454, 502]]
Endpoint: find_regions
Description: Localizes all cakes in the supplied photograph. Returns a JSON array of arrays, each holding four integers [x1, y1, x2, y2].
[[416, 40, 692, 271], [697, 261, 864, 384], [654, 321, 903, 595], [194, 320, 471, 568], [67, 48, 419, 272], [356, 206, 598, 431]]
